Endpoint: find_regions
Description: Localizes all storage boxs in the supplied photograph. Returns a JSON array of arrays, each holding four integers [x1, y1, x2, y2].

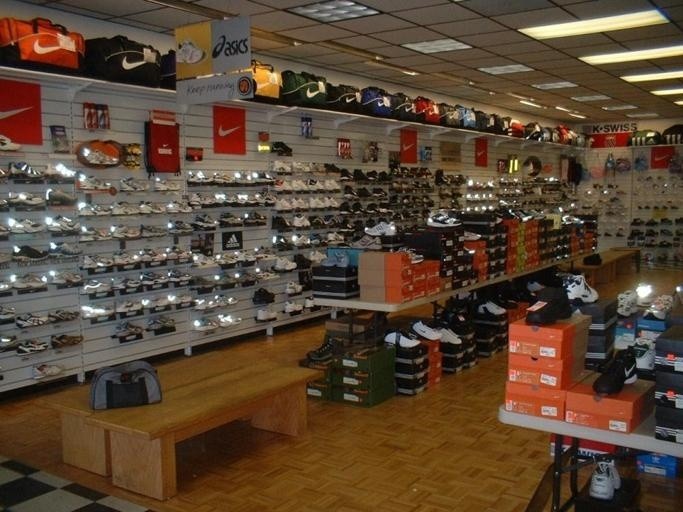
[[505, 303, 681, 480], [304, 209, 600, 409]]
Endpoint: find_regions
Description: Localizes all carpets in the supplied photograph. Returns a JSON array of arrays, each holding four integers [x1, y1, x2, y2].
[[0, 449, 157, 512]]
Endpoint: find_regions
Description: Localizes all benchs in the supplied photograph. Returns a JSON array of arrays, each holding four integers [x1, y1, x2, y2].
[[79, 353, 328, 503], [31, 346, 273, 479], [587, 248, 641, 284]]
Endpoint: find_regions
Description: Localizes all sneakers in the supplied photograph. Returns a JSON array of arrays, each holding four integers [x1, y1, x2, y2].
[[617, 290, 638, 317], [463, 231, 481, 242], [271, 141, 292, 153], [525, 287, 571, 324], [627, 216, 683, 247], [0, 135, 21, 152], [643, 295, 673, 320], [592, 337, 655, 394], [77, 176, 194, 337], [589, 459, 621, 500], [307, 337, 341, 361], [0, 162, 83, 380], [384, 320, 463, 348], [427, 213, 462, 227], [341, 169, 398, 250], [390, 167, 434, 233], [435, 169, 466, 209], [400, 247, 424, 264], [565, 276, 599, 304], [189, 171, 277, 332], [477, 280, 544, 316], [485, 207, 546, 224], [272, 161, 347, 313]]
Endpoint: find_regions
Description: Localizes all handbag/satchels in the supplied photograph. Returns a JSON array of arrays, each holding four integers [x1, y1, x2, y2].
[[605, 151, 649, 172], [83, 34, 162, 88], [224, 59, 283, 104], [90, 361, 162, 410], [0, 18, 85, 74], [326, 82, 363, 114], [159, 49, 197, 90], [360, 86, 392, 118], [629, 124, 683, 145], [280, 70, 328, 109], [391, 91, 416, 123], [414, 96, 584, 141]]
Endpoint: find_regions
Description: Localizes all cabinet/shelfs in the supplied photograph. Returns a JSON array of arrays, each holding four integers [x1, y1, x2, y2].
[[564, 169, 683, 266], [344, 170, 564, 225]]
